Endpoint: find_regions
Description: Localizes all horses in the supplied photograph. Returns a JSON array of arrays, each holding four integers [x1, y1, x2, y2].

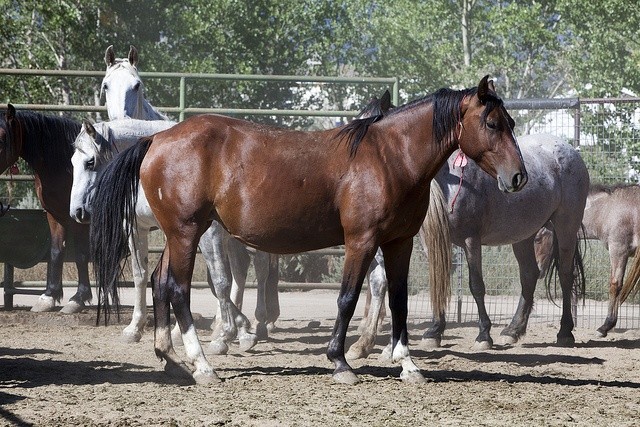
[[353, 90, 587, 351], [90, 45, 253, 352], [534, 179, 636, 347], [88, 73, 530, 387], [72, 111, 451, 368], [0, 100, 286, 344]]
